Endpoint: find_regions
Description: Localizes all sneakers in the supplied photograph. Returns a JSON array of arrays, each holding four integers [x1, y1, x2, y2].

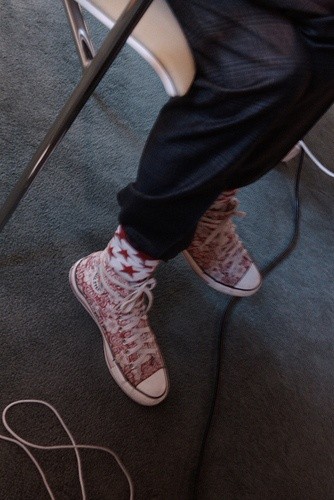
[[67, 252, 170, 405], [179, 190, 263, 298]]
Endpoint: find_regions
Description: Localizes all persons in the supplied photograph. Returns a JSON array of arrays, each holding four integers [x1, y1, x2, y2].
[[69, 0, 334, 410]]
